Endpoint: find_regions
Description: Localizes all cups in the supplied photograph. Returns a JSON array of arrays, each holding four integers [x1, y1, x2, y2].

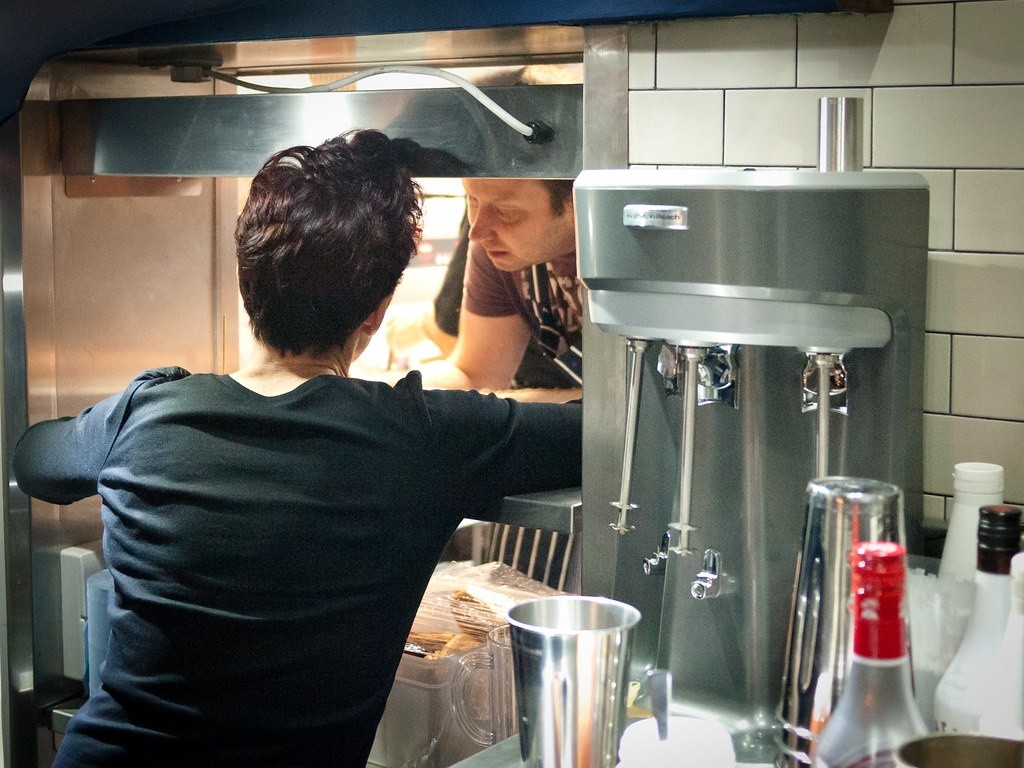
[[451, 625, 519, 746], [773, 476, 908, 768], [505, 595, 641, 768], [893, 734, 1024, 768]]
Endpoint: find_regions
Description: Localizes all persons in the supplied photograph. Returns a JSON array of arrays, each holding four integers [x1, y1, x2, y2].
[[11, 128, 583, 768], [422, 174, 582, 394]]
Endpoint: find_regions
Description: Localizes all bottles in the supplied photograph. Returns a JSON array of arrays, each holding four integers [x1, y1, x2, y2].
[[616, 717, 736, 768], [931, 462, 1024, 740], [811, 539, 928, 768]]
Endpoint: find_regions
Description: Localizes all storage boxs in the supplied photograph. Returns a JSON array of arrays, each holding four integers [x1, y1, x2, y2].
[[366, 638, 486, 767]]
